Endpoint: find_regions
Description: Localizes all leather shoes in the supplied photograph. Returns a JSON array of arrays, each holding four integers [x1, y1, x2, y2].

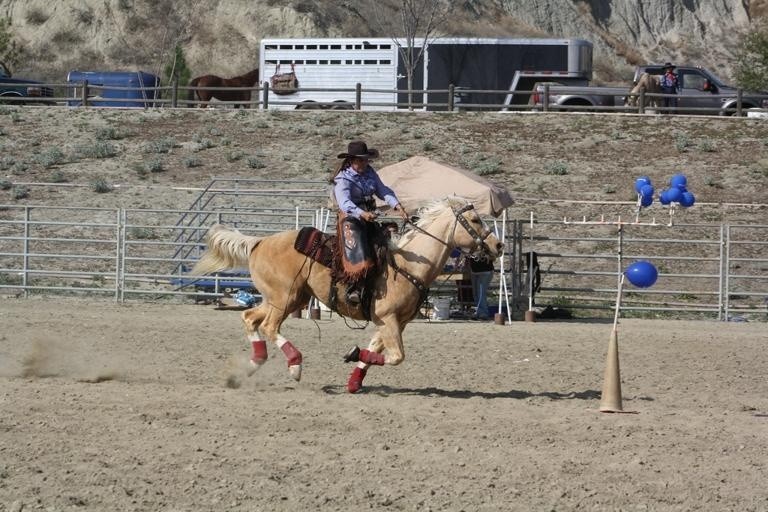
[[349, 290, 359, 303]]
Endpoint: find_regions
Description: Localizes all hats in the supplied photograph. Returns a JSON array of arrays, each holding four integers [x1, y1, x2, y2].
[[662, 62, 676, 69], [338, 143, 378, 158]]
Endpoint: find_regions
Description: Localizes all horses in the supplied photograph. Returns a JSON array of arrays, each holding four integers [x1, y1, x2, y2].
[[624, 72, 667, 115], [187, 192, 505, 396], [187, 68, 259, 109]]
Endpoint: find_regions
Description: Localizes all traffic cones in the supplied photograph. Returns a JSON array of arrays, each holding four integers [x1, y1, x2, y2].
[[587, 329, 638, 416]]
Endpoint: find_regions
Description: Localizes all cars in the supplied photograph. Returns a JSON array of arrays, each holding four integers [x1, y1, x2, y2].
[[0, 61, 56, 108]]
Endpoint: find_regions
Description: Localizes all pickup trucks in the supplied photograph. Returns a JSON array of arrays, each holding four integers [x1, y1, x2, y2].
[[532, 64, 767, 118]]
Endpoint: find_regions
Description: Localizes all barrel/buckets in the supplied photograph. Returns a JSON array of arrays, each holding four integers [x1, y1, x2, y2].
[[433, 299, 450, 320]]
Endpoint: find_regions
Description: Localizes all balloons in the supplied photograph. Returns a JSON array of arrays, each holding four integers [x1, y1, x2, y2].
[[634, 174, 695, 207], [624, 260, 658, 287]]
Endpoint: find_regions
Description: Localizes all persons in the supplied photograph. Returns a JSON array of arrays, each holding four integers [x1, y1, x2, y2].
[[468, 250, 495, 321], [660, 62, 680, 114], [328, 139, 410, 304]]
